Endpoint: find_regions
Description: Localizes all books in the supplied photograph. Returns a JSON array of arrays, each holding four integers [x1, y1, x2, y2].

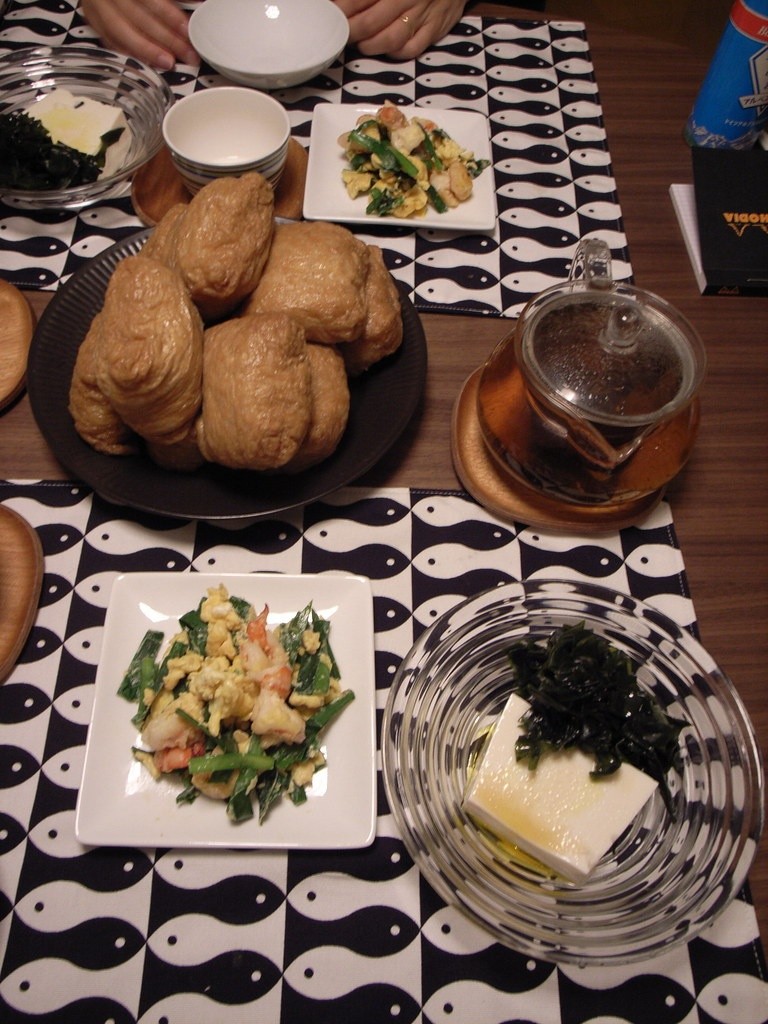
[[669, 146, 768, 296]]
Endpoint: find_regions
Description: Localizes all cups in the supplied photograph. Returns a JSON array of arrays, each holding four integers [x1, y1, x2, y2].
[[162, 87, 291, 197]]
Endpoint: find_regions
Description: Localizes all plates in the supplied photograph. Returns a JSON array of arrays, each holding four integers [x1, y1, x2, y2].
[[301, 100, 496, 231], [1, 503, 44, 684], [453, 362, 664, 533], [130, 136, 308, 232], [27, 217, 426, 520], [0, 276, 36, 410], [75, 572, 378, 850]]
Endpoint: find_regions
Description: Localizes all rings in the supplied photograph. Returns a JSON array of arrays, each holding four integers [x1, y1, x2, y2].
[[400, 15, 415, 39]]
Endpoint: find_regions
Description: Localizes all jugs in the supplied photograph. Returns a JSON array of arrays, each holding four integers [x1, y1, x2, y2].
[[475, 237, 708, 517]]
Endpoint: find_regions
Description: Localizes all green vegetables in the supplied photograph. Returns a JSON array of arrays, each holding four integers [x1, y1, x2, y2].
[[0, 107, 125, 191], [503, 618, 690, 824], [349, 119, 491, 217], [115, 598, 358, 822]]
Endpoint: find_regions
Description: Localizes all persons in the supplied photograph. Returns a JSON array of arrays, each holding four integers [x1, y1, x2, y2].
[[83, 0, 468, 69]]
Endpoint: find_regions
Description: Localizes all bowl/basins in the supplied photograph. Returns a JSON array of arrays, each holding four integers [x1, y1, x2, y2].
[[380, 578, 767, 965], [188, 0, 351, 89], [0, 46, 175, 207]]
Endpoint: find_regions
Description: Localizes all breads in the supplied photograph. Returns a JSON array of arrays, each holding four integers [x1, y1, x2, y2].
[[67, 171, 405, 477]]
